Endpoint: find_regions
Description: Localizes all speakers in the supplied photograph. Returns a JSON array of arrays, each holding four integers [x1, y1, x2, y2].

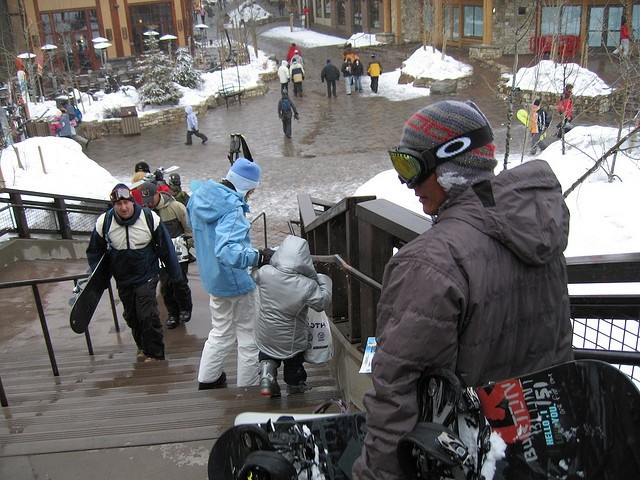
[[287, 379, 309, 395], [258, 360, 281, 395]]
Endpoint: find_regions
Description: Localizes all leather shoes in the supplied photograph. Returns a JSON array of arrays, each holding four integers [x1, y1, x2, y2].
[[379, 71, 382, 75], [322, 79, 324, 83], [367, 72, 370, 76], [192, 127, 197, 131], [258, 247, 276, 267], [337, 78, 339, 81]]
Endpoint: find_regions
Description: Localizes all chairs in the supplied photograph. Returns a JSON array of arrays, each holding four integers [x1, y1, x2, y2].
[[179, 309, 192, 322], [145, 355, 162, 363], [165, 314, 180, 328], [199, 371, 226, 389], [137, 348, 146, 357]]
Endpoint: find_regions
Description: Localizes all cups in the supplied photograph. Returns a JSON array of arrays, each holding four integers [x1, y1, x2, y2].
[[110, 189, 134, 201], [388, 126, 494, 189]]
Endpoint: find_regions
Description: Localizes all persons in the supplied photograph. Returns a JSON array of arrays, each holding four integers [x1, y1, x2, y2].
[[350, 100, 574, 480], [86, 184, 182, 362], [278, 90, 299, 138], [290, 50, 303, 66], [286, 43, 301, 63], [321, 60, 340, 98], [129, 162, 162, 207], [367, 53, 382, 93], [246, 234, 332, 397], [61, 99, 89, 150], [56, 108, 71, 138], [49, 112, 62, 137], [529, 98, 548, 155], [343, 43, 358, 85], [186, 158, 276, 392], [140, 184, 197, 329], [612, 18, 631, 60], [168, 173, 190, 207], [184, 106, 208, 145], [341, 58, 354, 95], [352, 57, 364, 93], [153, 169, 175, 196], [556, 84, 574, 138], [278, 60, 290, 94], [290, 58, 305, 97]]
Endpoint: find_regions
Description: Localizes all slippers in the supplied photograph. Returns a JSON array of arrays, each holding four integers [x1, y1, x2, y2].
[[74, 107, 82, 124], [281, 96, 290, 112]]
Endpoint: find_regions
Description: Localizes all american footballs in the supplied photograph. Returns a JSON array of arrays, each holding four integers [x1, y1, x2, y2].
[[208, 360, 640, 480], [17, 71, 30, 102], [69, 252, 113, 333], [157, 237, 189, 269], [128, 166, 180, 191], [516, 109, 546, 141]]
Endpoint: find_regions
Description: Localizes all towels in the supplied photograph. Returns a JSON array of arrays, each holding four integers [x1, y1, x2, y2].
[[26, 119, 37, 137], [121, 107, 141, 136], [35, 119, 49, 138]]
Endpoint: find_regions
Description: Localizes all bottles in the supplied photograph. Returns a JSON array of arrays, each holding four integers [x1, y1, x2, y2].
[[169, 173, 180, 184], [137, 182, 157, 202], [295, 50, 298, 53], [327, 60, 331, 64], [225, 158, 261, 199], [397, 101, 497, 196], [371, 53, 376, 56], [112, 184, 134, 205], [153, 170, 164, 181], [135, 162, 151, 173], [292, 58, 296, 61]]
